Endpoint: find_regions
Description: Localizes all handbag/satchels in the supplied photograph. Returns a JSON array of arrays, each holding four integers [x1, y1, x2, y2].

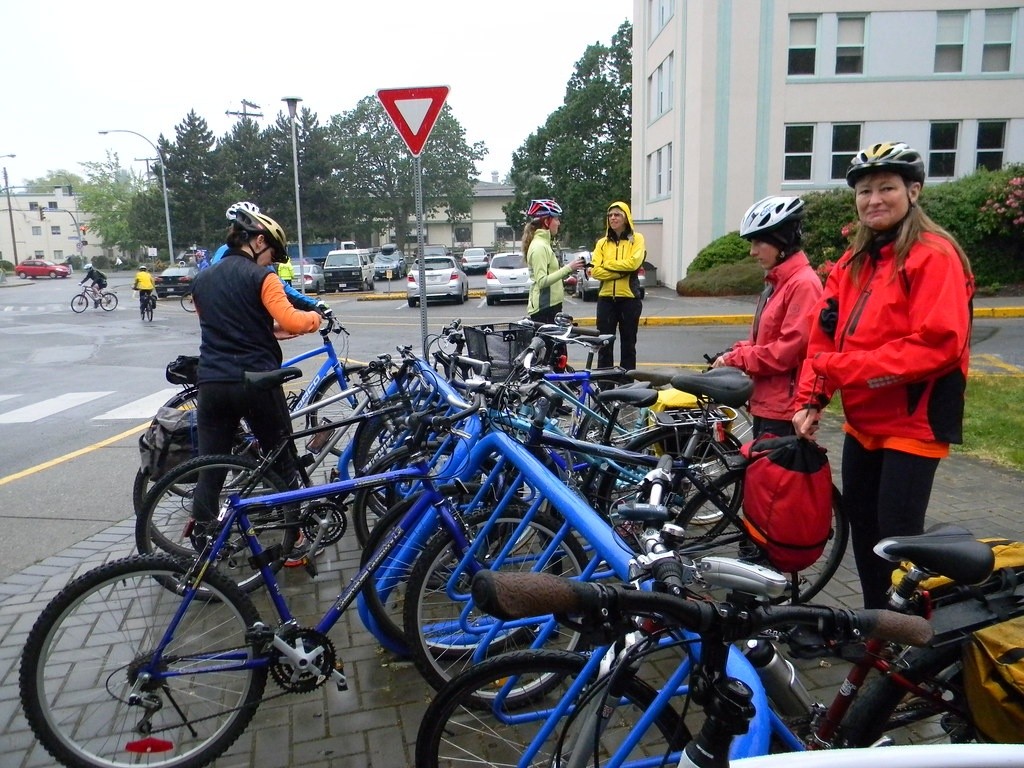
[[742, 437, 831, 575], [139, 407, 200, 483], [150, 295, 157, 309]]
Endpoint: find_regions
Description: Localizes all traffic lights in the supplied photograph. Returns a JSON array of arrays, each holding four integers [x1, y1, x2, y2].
[[81, 227, 86, 235]]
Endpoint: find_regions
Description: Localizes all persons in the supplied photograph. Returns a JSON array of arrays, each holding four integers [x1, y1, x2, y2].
[[590, 201, 646, 411], [80, 264, 107, 308], [523, 200, 586, 417], [115, 257, 123, 268], [66, 257, 74, 273], [134, 266, 155, 314], [809, 142, 974, 661], [711, 195, 823, 589], [191, 201, 331, 566]]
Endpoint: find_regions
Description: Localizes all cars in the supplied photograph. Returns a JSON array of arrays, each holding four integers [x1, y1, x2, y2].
[[288, 241, 646, 308], [155, 267, 200, 299]]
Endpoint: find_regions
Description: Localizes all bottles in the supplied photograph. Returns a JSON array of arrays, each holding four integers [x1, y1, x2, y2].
[[305, 416, 335, 454]]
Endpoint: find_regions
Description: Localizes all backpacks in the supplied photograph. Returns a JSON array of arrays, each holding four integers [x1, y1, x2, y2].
[[97, 269, 106, 279]]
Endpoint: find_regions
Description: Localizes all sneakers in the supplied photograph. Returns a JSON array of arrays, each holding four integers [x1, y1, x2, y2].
[[282, 528, 323, 567]]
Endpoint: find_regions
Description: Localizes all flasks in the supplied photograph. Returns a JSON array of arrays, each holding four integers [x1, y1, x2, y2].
[[742, 638, 813, 717]]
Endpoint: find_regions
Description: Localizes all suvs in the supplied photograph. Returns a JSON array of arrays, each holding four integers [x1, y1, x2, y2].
[[15, 259, 70, 279]]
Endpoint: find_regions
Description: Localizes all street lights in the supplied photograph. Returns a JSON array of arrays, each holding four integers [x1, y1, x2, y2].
[[279, 95, 307, 297], [98, 129, 174, 264]]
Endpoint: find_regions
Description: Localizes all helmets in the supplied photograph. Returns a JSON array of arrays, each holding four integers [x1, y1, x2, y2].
[[236, 207, 289, 264], [845, 141, 926, 189], [83, 263, 92, 270], [226, 201, 261, 220], [139, 265, 146, 270], [528, 200, 563, 216], [740, 196, 804, 239]]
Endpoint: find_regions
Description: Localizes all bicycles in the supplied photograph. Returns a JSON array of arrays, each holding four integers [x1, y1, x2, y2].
[[17, 279, 1024, 768]]
